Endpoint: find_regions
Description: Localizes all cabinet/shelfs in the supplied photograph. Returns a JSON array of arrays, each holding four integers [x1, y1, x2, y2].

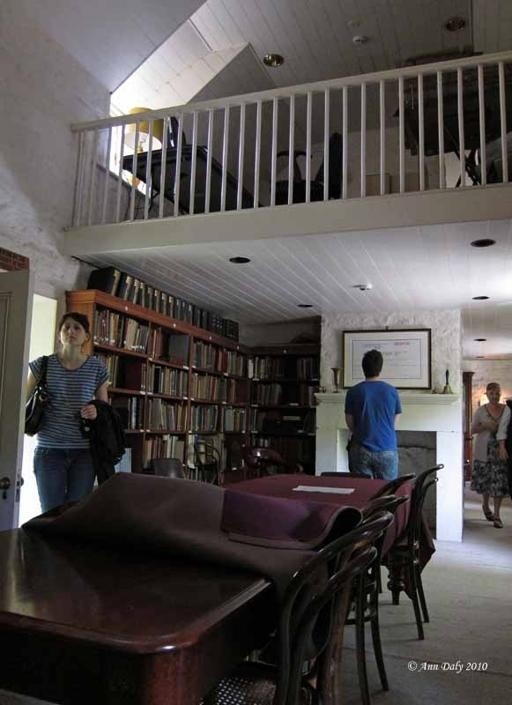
[[67, 290, 252, 483], [243, 345, 322, 474]]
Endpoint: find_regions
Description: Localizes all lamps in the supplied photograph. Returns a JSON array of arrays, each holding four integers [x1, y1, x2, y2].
[[124, 105, 164, 187]]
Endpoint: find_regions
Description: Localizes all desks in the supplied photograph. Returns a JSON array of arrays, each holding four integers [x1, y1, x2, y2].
[[393, 81, 512, 186], [121, 145, 264, 219], [0, 473, 413, 704]]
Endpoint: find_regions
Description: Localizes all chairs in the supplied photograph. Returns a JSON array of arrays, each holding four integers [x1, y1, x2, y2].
[[242, 444, 304, 475], [269, 132, 348, 206], [185, 460, 448, 704], [149, 456, 186, 481]]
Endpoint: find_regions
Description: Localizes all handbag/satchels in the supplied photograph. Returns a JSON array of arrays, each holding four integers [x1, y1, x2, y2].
[[24, 354, 50, 437]]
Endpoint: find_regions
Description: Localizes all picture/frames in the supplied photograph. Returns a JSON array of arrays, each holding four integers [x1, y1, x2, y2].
[[341, 326, 434, 389]]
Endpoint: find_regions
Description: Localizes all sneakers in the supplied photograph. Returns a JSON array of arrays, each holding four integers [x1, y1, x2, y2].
[[493, 518, 504, 529], [481, 503, 495, 521]]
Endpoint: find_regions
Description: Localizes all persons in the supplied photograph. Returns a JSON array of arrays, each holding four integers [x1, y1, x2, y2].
[[470, 382, 510, 529], [344, 350, 403, 482], [22, 310, 110, 512]]
